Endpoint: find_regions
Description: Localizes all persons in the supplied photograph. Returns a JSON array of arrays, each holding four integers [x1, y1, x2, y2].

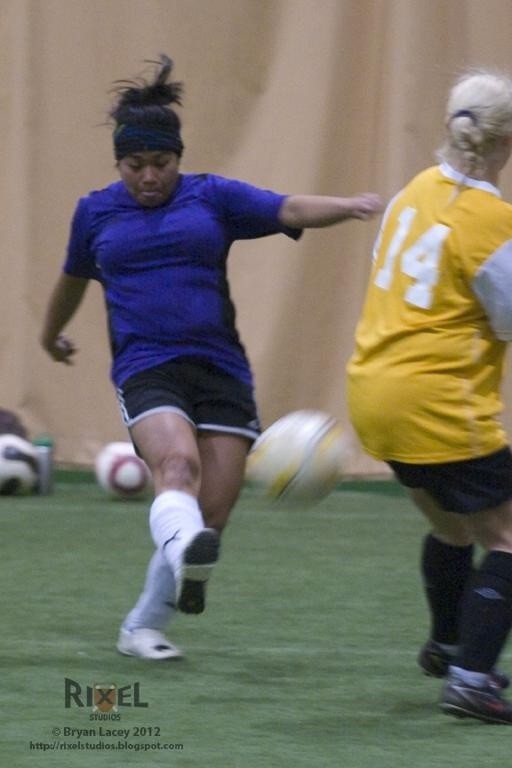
[[37, 57, 385, 663], [342, 71, 512, 725]]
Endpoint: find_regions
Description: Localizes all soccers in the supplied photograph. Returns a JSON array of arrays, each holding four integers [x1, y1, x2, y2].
[[97, 441, 152, 498], [0, 434, 40, 496], [248, 409, 351, 509]]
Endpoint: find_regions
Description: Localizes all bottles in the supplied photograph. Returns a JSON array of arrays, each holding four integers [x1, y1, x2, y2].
[[33, 436, 53, 498]]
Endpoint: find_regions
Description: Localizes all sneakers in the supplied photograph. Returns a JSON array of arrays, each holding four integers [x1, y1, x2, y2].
[[175, 527, 220, 614], [439, 673, 512, 725], [418, 637, 509, 688], [116, 622, 185, 662]]
